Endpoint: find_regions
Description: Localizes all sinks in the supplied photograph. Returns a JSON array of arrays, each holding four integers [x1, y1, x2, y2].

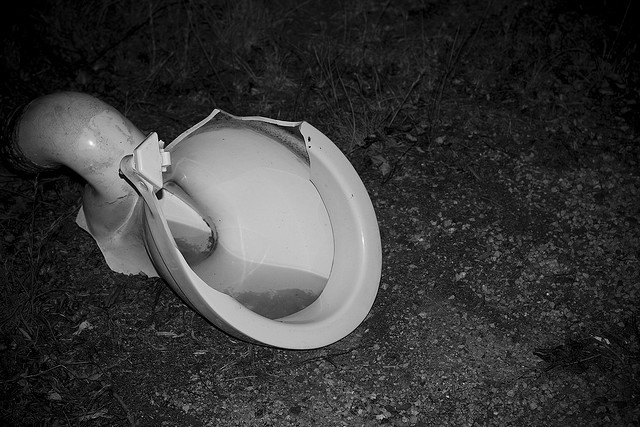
[[1, 91, 384, 351]]
